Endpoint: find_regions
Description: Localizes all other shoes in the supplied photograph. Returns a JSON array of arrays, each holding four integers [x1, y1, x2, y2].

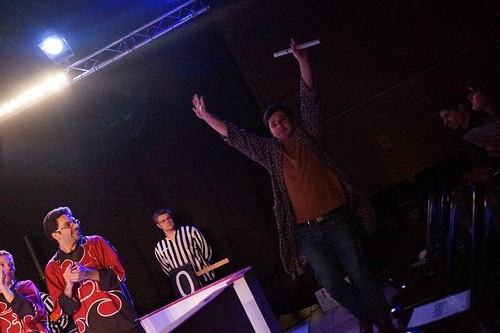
[[359, 318, 373, 333], [378, 319, 396, 333]]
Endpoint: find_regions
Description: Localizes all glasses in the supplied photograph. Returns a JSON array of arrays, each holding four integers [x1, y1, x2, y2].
[[61, 219, 80, 230], [158, 217, 171, 223]]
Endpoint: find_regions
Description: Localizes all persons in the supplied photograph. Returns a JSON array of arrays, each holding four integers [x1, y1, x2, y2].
[[0, 250, 46, 333], [44, 206, 146, 333], [409, 80, 500, 267], [192, 37, 399, 333], [153, 208, 216, 286], [39, 292, 69, 333]]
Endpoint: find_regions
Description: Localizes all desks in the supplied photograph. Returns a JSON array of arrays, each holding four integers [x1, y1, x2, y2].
[[135, 263, 281, 333]]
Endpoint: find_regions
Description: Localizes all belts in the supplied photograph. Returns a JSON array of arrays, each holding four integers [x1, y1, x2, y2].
[[298, 206, 340, 228]]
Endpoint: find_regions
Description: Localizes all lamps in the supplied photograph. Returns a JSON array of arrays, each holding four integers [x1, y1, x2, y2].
[[37, 30, 75, 65]]
